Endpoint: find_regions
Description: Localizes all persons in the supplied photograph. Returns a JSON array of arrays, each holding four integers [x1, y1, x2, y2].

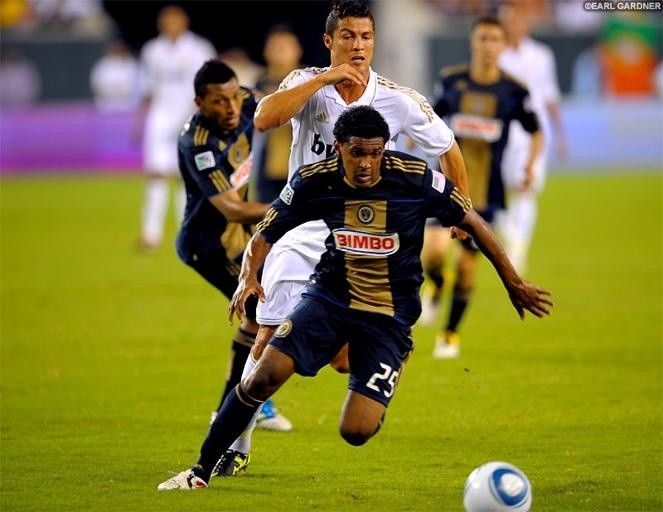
[[175, 60, 294, 437], [400, 17, 544, 363], [155, 105, 555, 491], [0, 0, 662, 273], [210, 1, 472, 475]]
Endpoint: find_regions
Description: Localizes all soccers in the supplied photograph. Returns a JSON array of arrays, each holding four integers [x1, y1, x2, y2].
[[462, 461, 531, 512]]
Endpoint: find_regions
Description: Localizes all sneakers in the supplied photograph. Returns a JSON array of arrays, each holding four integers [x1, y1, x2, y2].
[[157, 399, 291, 490], [433, 330, 458, 359], [419, 283, 445, 322]]
